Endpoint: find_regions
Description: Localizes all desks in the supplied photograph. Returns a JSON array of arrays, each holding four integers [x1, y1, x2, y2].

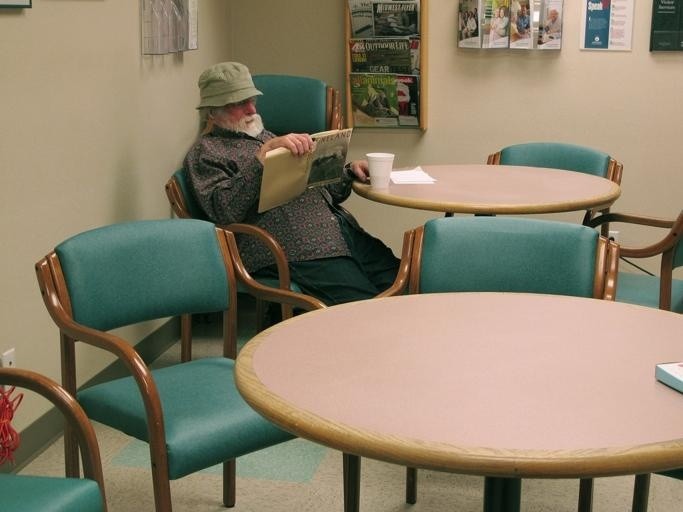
[[235, 290, 683, 512], [351, 163, 621, 236]]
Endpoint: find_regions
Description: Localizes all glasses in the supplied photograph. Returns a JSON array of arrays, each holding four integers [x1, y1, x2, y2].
[[235, 97, 257, 106]]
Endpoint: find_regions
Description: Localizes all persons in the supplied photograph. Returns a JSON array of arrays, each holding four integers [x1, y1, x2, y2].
[[182, 61, 402, 333], [459, 8, 479, 40], [492, 6, 509, 36], [518, 5, 529, 33], [546, 9, 561, 33], [511, 15, 528, 40]]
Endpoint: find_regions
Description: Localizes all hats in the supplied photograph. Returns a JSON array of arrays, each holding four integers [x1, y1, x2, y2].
[[196, 62, 264, 109]]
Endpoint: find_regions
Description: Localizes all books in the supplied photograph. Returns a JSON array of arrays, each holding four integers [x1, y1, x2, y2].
[[257, 128, 353, 214], [483, 0, 509, 49], [350, 76, 419, 128], [349, 1, 420, 38], [349, 39, 421, 75], [509, 0, 534, 49], [458, 0, 482, 50], [537, 1, 563, 50]]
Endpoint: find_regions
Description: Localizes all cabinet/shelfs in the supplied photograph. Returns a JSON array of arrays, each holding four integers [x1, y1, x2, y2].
[[346, 2, 426, 129]]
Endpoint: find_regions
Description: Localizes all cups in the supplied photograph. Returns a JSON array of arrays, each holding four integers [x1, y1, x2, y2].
[[365, 153, 395, 192]]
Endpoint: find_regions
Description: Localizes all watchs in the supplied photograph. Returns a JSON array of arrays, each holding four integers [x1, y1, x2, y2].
[[345, 162, 354, 177]]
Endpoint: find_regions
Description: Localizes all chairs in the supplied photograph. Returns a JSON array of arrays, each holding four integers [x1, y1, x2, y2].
[[616, 211, 683, 312], [409, 219, 621, 304], [246, 78, 341, 136], [166, 160, 331, 363], [35, 218, 332, 511], [0, 371, 106, 507], [485, 141, 624, 234]]
